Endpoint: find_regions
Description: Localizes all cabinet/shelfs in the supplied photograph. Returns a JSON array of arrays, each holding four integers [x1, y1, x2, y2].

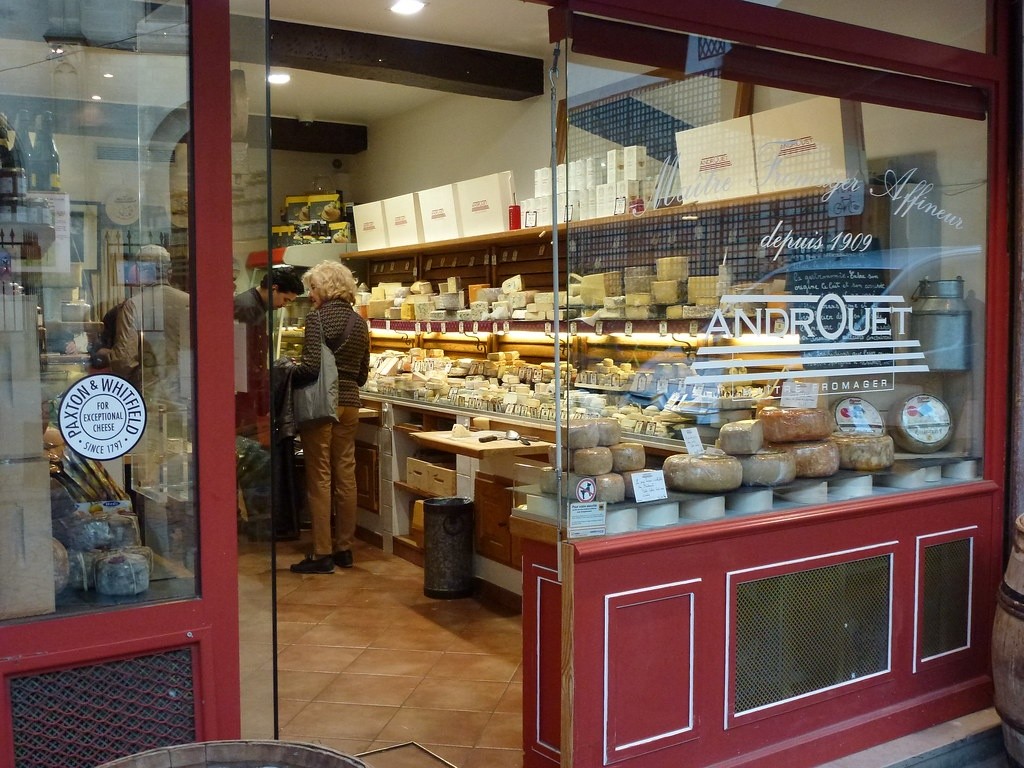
[[351, 439, 380, 515], [472, 470, 522, 572], [390, 403, 464, 568]]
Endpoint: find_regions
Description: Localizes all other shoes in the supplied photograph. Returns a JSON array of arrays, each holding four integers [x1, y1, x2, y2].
[[305, 549, 353, 568], [290, 555, 335, 575]]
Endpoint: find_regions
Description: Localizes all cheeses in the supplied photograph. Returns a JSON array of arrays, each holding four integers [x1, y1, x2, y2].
[[341, 254, 954, 504]]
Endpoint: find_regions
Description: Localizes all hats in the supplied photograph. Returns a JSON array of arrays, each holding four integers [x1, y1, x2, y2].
[[137, 245, 171, 273]]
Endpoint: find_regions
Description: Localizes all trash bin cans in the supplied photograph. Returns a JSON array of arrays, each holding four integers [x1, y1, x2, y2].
[[423, 496, 473, 600], [294, 454, 335, 530]]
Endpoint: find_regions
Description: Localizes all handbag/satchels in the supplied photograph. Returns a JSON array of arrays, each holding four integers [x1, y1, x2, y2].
[[290, 344, 340, 429]]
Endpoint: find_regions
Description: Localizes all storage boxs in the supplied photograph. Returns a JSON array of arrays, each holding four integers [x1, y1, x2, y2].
[[406, 456, 457, 550], [0, 294, 44, 461], [0, 457, 55, 621], [76, 492, 131, 514], [271, 194, 361, 248], [352, 95, 870, 251]]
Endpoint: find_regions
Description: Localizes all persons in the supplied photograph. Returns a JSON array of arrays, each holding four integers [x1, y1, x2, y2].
[[233, 267, 305, 541], [45, 321, 78, 356], [274, 260, 370, 575], [89, 244, 197, 562]]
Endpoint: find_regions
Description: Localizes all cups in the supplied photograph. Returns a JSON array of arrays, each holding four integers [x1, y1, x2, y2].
[[508, 205, 522, 230]]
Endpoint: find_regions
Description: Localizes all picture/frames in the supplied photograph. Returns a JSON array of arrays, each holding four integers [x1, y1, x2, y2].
[[69, 201, 101, 274]]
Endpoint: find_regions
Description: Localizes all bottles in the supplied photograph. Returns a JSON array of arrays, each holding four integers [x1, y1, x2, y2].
[[0, 118, 11, 197], [30, 111, 61, 189], [9, 108, 35, 196]]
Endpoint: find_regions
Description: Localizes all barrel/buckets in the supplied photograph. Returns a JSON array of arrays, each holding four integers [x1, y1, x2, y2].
[[990, 513, 1024, 766], [908, 280, 973, 368]]
